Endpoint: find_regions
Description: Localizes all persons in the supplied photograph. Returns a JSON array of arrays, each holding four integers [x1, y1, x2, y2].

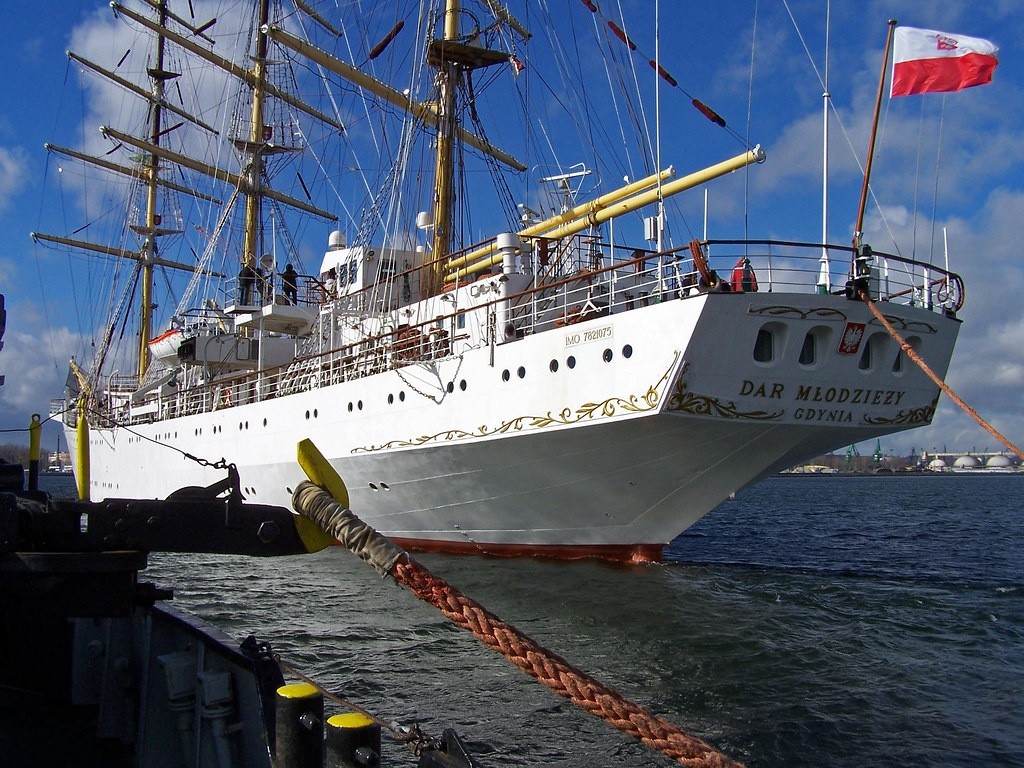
[[324, 272, 337, 301], [731, 257, 760, 293], [282, 265, 298, 306], [237, 266, 255, 306]]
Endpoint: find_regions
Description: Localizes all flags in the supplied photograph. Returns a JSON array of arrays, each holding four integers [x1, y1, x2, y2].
[[509, 56, 527, 76], [887, 27, 998, 98]]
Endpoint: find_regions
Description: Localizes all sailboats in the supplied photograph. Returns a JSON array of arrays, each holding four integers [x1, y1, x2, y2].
[[30, 1, 963, 571]]
